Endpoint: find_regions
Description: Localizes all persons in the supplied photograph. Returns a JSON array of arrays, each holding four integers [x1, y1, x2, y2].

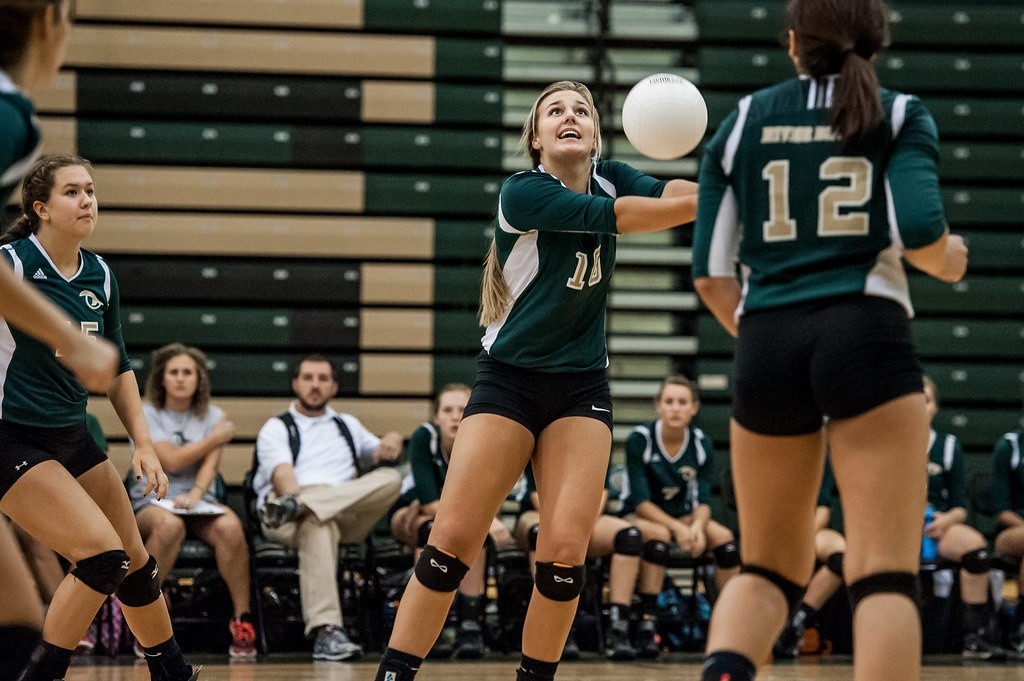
[[622, 372, 742, 654], [771, 367, 1023, 664], [0, 2, 197, 681], [688, 1, 970, 681], [387, 380, 642, 664], [373, 79, 701, 681], [121, 343, 269, 663], [253, 351, 406, 661]]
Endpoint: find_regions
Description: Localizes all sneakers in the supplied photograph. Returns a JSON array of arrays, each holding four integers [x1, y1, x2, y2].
[[563, 636, 580, 658], [605, 628, 635, 660], [312, 623, 362, 660], [450, 626, 484, 661], [260, 497, 296, 528], [229, 620, 258, 658]]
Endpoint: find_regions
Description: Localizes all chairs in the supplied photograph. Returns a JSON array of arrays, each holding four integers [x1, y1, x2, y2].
[[73, 452, 1024, 663]]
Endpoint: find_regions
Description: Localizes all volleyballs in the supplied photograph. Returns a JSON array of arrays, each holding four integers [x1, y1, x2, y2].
[[621, 73, 709, 163]]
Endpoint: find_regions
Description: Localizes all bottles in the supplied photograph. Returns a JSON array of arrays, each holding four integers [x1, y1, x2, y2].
[[916, 506, 939, 563]]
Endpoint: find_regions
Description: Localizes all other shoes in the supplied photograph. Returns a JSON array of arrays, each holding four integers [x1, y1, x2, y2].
[[776, 626, 798, 660], [634, 620, 659, 656], [961, 638, 994, 658]]
